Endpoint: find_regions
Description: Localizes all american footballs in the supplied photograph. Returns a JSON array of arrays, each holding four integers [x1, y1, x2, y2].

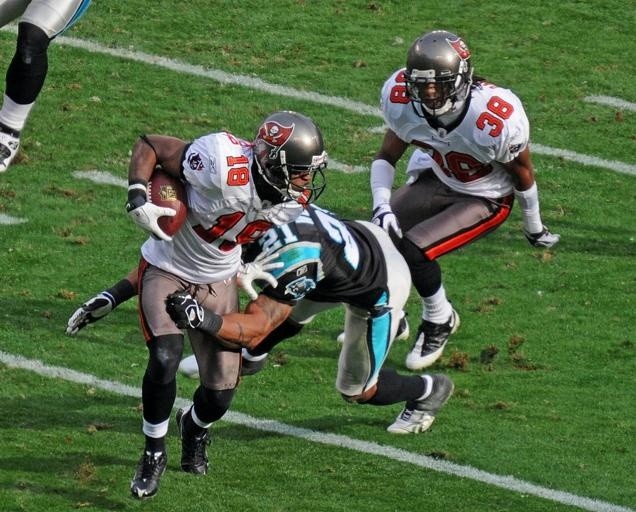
[[146, 166, 188, 238]]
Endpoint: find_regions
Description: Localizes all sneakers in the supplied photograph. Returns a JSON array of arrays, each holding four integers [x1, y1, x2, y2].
[[405, 308, 460, 371], [386, 374, 454, 434], [0, 122, 19, 173], [177, 348, 268, 380], [175, 404, 210, 475], [130, 450, 167, 501], [336, 312, 409, 344]]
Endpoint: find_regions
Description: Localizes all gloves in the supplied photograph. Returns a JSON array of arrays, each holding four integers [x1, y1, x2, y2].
[[236, 247, 284, 301], [522, 225, 561, 249], [64, 290, 116, 337], [123, 195, 176, 242], [165, 289, 204, 330], [370, 204, 403, 240]]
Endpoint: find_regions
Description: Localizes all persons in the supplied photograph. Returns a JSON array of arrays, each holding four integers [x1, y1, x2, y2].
[[0, 0, 92, 177], [334, 28, 562, 374], [64, 194, 457, 437], [124, 108, 329, 501]]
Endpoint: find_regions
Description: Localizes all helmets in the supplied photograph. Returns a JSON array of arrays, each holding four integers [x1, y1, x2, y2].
[[253, 110, 328, 206], [402, 30, 473, 119]]
